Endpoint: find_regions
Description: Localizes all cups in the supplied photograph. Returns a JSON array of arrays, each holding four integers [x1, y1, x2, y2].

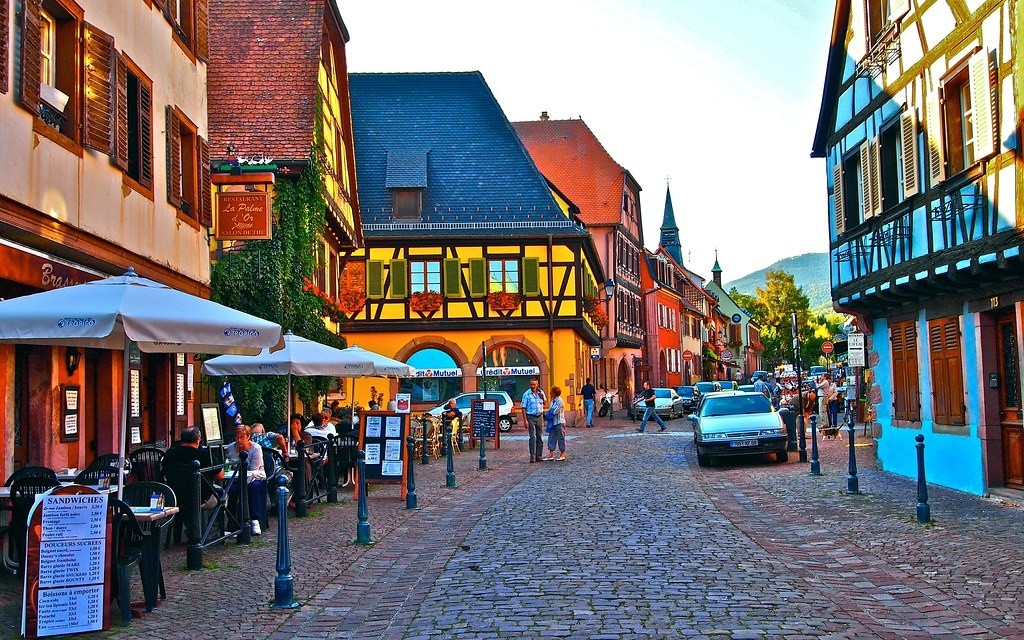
[[291, 447, 295, 453]]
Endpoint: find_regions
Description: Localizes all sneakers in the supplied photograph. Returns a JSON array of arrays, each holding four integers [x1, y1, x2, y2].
[[224, 530, 238, 538], [251, 519, 261, 535]]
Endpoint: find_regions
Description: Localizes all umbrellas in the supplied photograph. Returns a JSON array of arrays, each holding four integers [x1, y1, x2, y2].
[[0, 266, 286, 514], [339, 340, 417, 431], [202, 328, 376, 454]]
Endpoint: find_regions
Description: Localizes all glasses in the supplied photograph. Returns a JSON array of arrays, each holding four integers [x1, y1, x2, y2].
[[323, 416, 326, 420], [234, 434, 247, 440], [290, 414, 301, 418]]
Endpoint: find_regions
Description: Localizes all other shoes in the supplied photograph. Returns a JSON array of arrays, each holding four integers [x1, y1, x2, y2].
[[543, 457, 554, 461], [530, 454, 542, 462], [818, 422, 832, 429], [832, 425, 837, 427], [556, 456, 565, 461], [636, 428, 644, 433], [658, 427, 667, 432], [798, 433, 811, 439], [587, 424, 593, 428]]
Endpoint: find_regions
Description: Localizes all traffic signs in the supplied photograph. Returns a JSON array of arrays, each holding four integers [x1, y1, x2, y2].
[[848, 334, 865, 367]]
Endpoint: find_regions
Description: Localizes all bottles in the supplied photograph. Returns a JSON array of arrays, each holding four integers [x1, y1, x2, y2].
[[97, 470, 110, 489], [224, 457, 230, 471]]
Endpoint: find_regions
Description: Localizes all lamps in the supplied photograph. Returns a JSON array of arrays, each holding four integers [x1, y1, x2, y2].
[[66, 347, 83, 375], [989, 373, 998, 387], [718, 331, 722, 337], [598, 279, 615, 301]]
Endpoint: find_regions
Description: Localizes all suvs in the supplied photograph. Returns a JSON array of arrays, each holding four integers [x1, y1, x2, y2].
[[810, 366, 827, 376], [694, 381, 722, 395]]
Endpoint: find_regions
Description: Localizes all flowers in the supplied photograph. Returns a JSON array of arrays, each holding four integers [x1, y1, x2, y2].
[[486, 292, 524, 309], [339, 290, 368, 312], [409, 291, 443, 311], [584, 294, 608, 330]]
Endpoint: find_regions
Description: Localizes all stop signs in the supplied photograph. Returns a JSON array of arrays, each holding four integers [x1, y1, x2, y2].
[[821, 341, 834, 353], [682, 350, 693, 361]]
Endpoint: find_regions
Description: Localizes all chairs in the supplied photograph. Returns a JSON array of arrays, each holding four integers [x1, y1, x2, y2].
[[2, 409, 473, 624]]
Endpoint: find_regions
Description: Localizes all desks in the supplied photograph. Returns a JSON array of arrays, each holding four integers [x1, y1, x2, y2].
[[223, 469, 254, 479], [0, 484, 125, 498], [109, 505, 179, 520], [55, 470, 129, 478], [288, 450, 309, 459]]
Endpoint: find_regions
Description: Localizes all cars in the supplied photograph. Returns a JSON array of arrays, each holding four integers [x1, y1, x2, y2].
[[672, 386, 704, 411], [738, 385, 773, 404], [719, 381, 739, 392], [629, 388, 683, 420], [687, 391, 790, 466], [423, 391, 517, 432], [771, 379, 818, 409], [794, 367, 803, 372], [751, 371, 768, 384]]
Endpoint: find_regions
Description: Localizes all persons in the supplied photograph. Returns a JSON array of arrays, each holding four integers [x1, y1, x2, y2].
[[581, 378, 599, 429], [633, 381, 666, 433], [166, 378, 566, 540], [732, 368, 839, 440]]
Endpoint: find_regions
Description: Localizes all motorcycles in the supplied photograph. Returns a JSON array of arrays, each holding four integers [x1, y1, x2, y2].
[[598, 389, 619, 417]]
